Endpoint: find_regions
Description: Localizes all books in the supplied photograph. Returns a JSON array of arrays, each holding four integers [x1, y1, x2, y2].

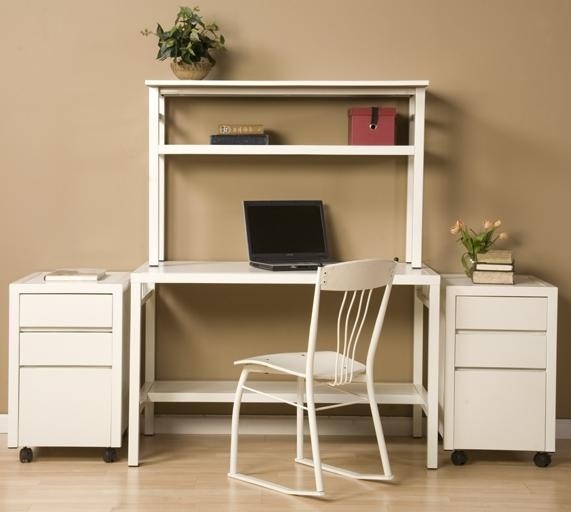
[[471, 248, 515, 285], [43, 267, 106, 281], [210, 134, 272, 145], [216, 123, 264, 135]]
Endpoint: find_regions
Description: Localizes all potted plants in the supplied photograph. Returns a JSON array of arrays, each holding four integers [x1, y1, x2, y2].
[[140, 3, 229, 80]]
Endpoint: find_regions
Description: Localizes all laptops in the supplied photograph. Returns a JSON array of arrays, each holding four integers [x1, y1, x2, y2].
[[244, 201, 335, 270]]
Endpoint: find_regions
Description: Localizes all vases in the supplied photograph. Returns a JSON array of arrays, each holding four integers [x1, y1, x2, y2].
[[462, 252, 477, 277], [171, 61, 212, 80]]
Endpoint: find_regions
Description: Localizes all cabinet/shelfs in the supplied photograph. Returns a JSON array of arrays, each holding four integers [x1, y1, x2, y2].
[[431, 273, 559, 466], [146, 80, 431, 265], [10, 272, 132, 461]]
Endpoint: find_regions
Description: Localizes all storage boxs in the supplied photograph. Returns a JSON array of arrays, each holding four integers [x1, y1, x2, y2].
[[347, 107, 397, 146]]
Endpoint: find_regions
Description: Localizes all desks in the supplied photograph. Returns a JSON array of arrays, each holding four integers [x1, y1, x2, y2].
[[128, 259, 441, 469]]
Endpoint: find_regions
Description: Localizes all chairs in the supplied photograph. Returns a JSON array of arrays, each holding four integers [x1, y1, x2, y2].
[[228, 258, 398, 500]]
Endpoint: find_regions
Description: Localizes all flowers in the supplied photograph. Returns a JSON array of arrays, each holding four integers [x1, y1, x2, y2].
[[449, 216, 507, 279]]
